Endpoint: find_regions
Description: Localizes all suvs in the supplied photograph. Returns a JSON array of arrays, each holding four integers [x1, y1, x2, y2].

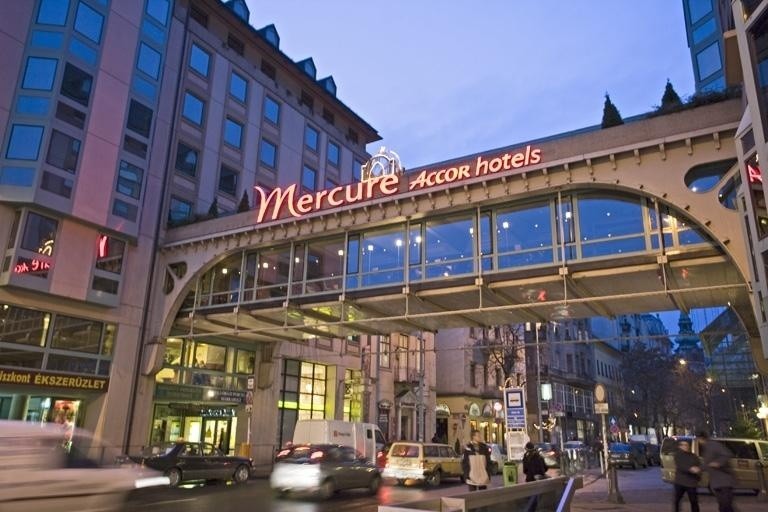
[[380, 442, 464, 487]]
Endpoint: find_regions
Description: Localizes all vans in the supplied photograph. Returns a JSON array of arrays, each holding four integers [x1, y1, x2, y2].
[[659, 435, 768, 496]]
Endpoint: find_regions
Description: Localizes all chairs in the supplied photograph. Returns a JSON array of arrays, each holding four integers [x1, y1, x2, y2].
[[188, 449, 197, 456]]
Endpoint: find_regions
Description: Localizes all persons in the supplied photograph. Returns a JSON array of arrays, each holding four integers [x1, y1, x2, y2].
[[673, 439, 701, 511], [461, 430, 492, 512], [432, 433, 443, 443], [694, 430, 738, 511], [522, 441, 549, 497]]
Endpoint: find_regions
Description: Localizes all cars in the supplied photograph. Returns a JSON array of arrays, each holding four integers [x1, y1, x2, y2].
[[268, 443, 381, 501], [113, 442, 256, 489], [0, 419, 170, 512], [534, 441, 661, 470], [485, 443, 507, 476]]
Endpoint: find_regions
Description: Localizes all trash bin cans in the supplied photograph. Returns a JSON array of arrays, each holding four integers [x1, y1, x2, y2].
[[503, 463, 518, 488]]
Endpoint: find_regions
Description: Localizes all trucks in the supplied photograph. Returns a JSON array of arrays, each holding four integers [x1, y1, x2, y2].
[[292, 419, 387, 457]]
